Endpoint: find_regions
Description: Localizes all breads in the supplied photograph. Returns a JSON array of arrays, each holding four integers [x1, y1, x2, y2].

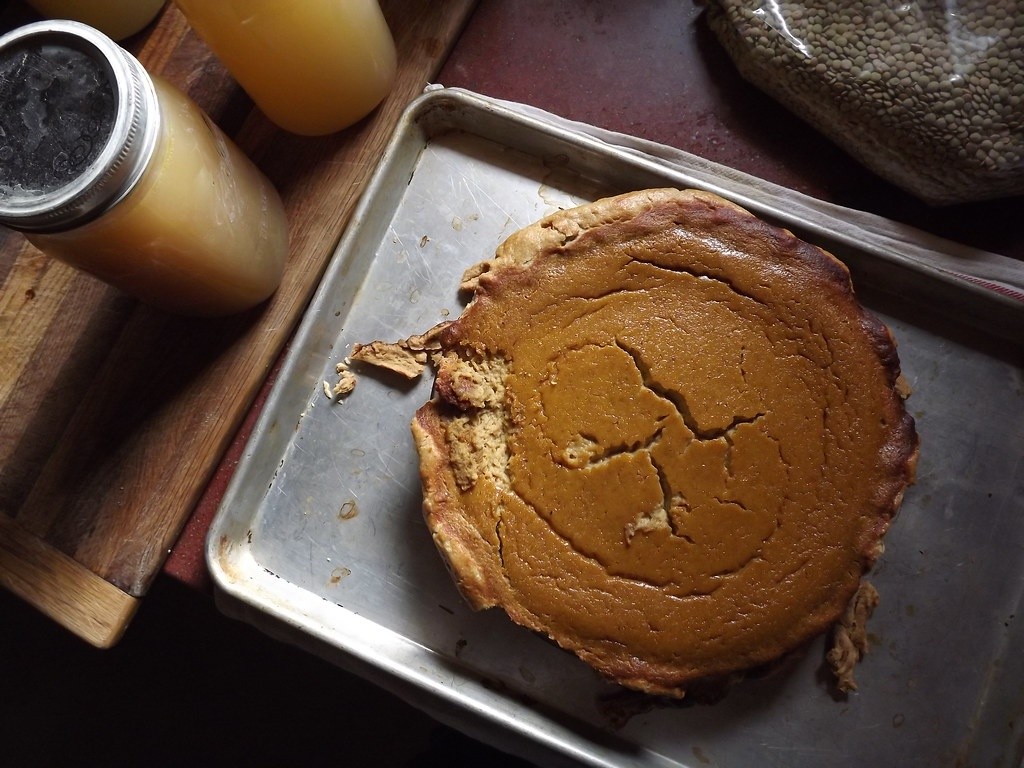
[[350, 188, 919, 705]]
